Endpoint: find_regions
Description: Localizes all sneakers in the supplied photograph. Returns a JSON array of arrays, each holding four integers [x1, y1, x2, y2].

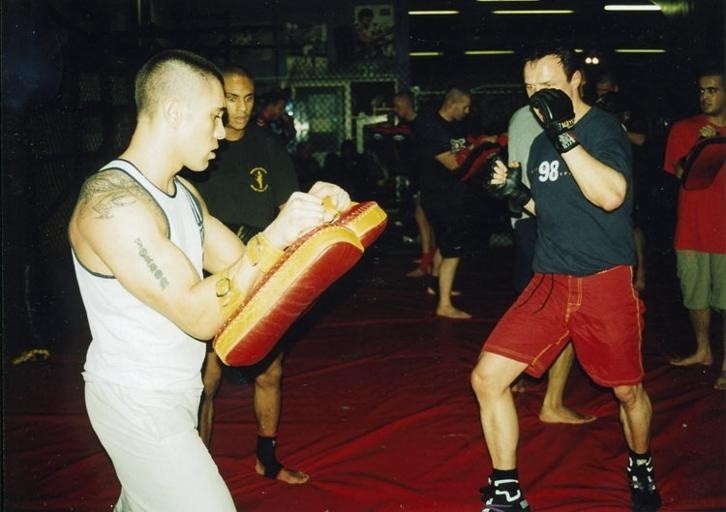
[[480, 473, 531, 512], [626, 463, 664, 511]]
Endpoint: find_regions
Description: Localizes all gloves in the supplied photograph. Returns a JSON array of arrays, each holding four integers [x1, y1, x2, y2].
[[269, 191, 330, 246], [483, 156, 532, 217], [307, 179, 353, 216], [528, 88, 581, 153]]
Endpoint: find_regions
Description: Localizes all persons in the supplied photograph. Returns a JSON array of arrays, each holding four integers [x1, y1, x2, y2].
[[661, 69, 726, 391], [63, 48, 352, 512], [192, 61, 312, 486], [353, 7, 395, 59], [469, 38, 661, 512]]
